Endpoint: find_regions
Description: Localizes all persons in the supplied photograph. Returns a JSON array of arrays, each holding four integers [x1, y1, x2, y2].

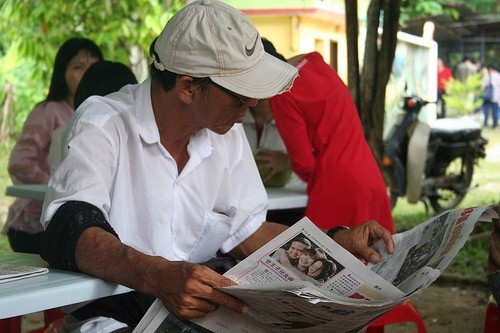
[[240, 97, 288, 153], [273, 237, 337, 286], [254, 35, 399, 242], [437, 52, 500, 128], [5, 37, 102, 257], [48, 60, 138, 177], [45, 0, 397, 332]]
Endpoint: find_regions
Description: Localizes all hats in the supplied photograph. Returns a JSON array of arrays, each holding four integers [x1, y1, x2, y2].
[[154, 0, 298, 99]]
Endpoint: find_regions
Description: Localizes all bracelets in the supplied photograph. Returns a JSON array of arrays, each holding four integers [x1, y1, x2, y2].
[[327, 225, 351, 238]]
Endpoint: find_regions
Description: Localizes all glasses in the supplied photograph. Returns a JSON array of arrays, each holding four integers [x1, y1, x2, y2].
[[214, 83, 249, 107]]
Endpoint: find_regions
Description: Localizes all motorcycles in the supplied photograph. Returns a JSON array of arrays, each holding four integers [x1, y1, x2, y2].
[[380, 81, 489, 217]]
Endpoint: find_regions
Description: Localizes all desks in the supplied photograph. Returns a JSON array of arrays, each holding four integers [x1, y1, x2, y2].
[[0, 252, 134, 333], [5, 178, 308, 210]]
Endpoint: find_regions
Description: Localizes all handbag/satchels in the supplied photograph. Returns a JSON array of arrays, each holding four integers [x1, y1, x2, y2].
[[479, 82, 493, 100]]
